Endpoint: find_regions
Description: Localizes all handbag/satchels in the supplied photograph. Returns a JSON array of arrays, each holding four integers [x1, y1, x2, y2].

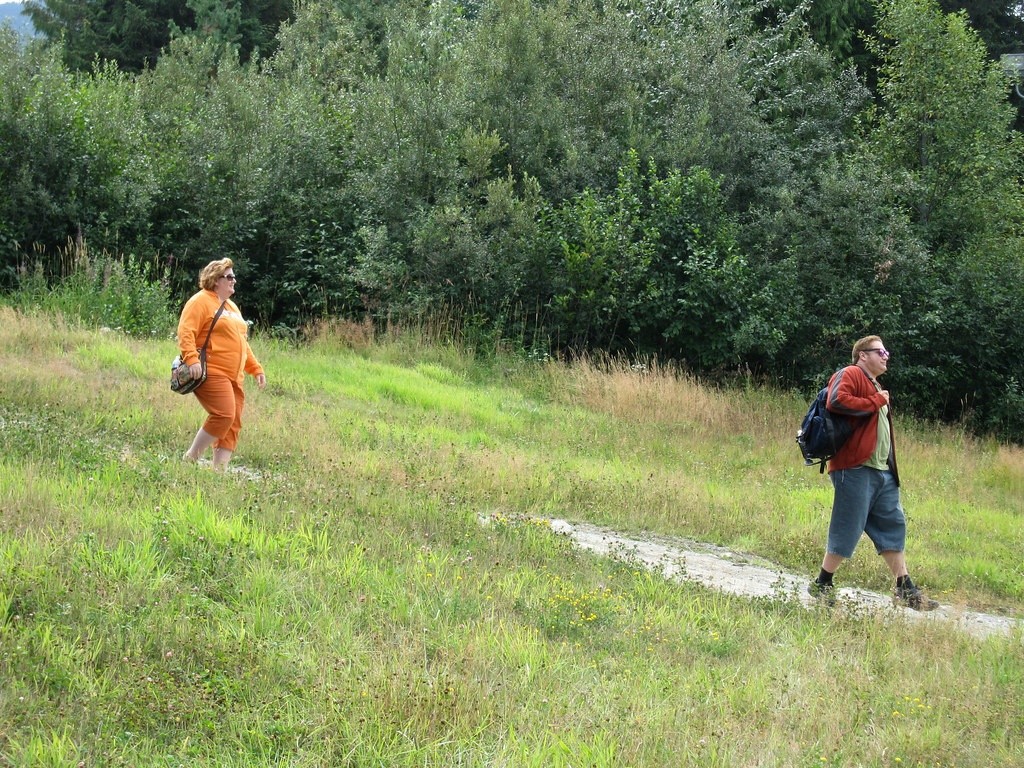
[[170, 348, 207, 395], [796, 365, 878, 474]]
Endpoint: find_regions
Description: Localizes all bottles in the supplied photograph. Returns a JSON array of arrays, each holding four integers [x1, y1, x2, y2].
[[172, 356, 181, 369], [797, 430, 811, 459]]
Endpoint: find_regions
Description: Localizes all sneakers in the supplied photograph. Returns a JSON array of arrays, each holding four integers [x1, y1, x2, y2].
[[894, 584, 939, 611], [807, 578, 835, 606]]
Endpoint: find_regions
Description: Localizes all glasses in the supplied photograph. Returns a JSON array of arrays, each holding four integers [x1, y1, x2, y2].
[[218, 273, 235, 281], [860, 348, 890, 357]]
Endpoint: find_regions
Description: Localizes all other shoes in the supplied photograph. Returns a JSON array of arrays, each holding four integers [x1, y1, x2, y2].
[[183, 454, 194, 463]]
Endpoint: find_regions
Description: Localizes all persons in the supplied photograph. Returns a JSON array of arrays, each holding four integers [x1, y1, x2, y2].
[[177, 258, 266, 468], [807, 335, 939, 612]]
